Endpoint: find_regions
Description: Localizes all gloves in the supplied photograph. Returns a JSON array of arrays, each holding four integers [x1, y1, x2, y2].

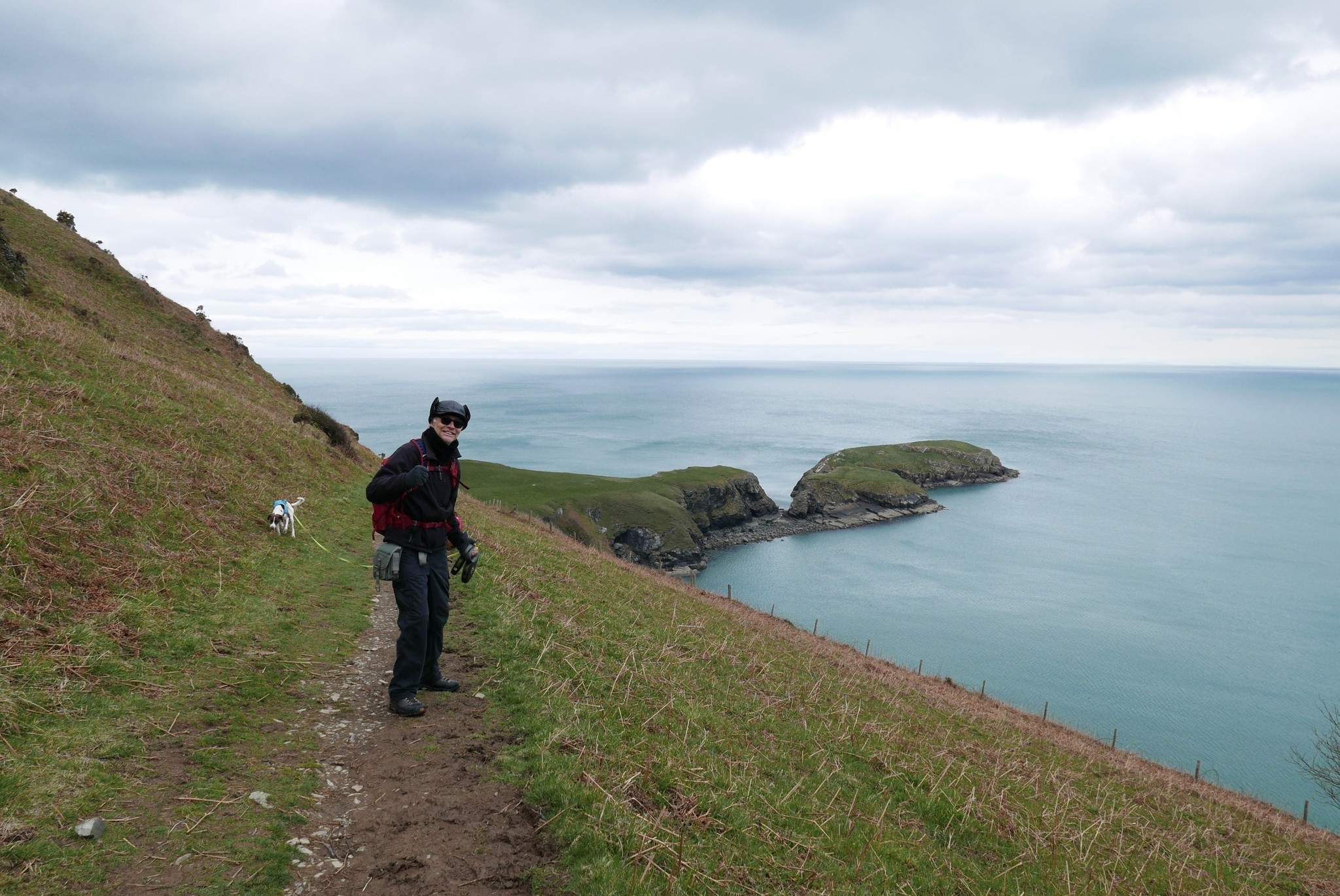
[[404, 465, 428, 489]]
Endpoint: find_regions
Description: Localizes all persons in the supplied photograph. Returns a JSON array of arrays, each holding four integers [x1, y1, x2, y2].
[[366, 398, 480, 717]]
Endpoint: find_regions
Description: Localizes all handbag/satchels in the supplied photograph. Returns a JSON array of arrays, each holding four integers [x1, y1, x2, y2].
[[373, 543, 402, 581]]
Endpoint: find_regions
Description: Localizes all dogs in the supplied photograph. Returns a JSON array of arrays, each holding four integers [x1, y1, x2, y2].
[[267, 497, 305, 538]]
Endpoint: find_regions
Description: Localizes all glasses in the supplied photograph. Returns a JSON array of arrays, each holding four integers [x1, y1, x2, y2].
[[437, 415, 464, 428]]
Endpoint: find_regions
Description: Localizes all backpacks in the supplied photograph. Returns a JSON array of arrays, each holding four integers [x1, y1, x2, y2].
[[372, 438, 458, 536]]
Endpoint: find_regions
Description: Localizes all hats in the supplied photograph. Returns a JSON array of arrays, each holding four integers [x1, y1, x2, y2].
[[428, 397, 470, 431]]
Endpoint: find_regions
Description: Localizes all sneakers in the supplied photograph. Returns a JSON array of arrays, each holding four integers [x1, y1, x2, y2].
[[419, 677, 459, 692], [389, 695, 426, 716]]
